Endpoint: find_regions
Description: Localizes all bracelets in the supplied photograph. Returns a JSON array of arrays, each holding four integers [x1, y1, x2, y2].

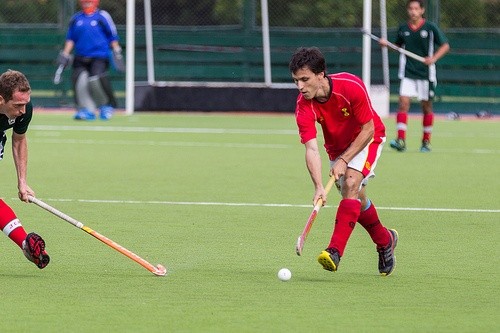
[[337, 157, 349, 166]]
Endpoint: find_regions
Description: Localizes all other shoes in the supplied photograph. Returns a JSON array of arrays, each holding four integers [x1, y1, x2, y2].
[[420, 140, 431, 152], [98, 102, 114, 119], [74, 105, 94, 119], [389, 139, 405, 152]]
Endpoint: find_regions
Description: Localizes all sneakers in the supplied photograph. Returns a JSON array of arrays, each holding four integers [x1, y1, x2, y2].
[[317, 247, 343, 271], [23, 232, 51, 269], [375, 228, 398, 276]]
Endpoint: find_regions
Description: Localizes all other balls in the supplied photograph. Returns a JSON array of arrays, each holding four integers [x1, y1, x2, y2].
[[277, 268, 293, 282]]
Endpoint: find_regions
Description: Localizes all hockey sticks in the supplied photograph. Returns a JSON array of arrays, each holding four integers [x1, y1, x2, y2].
[[27, 195, 167, 277], [360, 28, 426, 63], [295, 174, 336, 257], [53, 64, 64, 85]]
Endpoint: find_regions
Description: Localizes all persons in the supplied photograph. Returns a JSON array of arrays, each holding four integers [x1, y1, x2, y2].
[[290, 47, 399, 277], [57, 0, 126, 119], [0, 69, 51, 268], [379, 0, 451, 153]]
